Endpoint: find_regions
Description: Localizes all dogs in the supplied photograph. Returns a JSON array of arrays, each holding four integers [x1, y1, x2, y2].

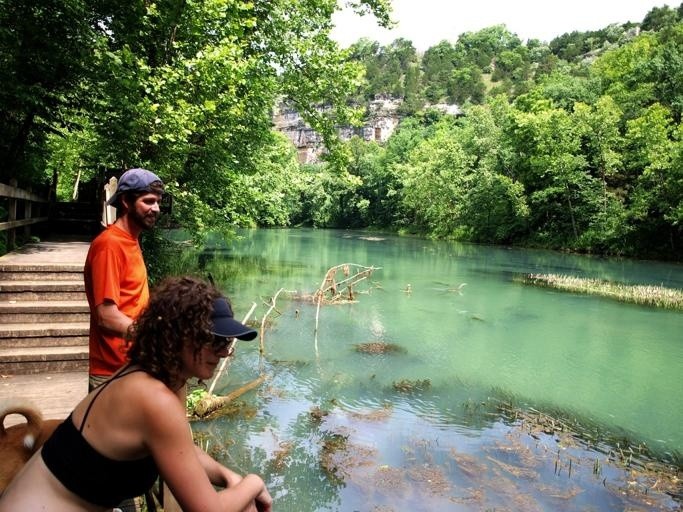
[[0, 398, 66, 498]]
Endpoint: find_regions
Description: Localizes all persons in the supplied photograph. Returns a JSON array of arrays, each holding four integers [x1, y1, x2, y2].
[[0, 275, 274, 511], [81, 166, 164, 389]]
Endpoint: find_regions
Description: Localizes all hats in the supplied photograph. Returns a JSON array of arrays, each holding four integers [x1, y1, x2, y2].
[[183, 299, 257, 342], [105, 169, 164, 209]]
